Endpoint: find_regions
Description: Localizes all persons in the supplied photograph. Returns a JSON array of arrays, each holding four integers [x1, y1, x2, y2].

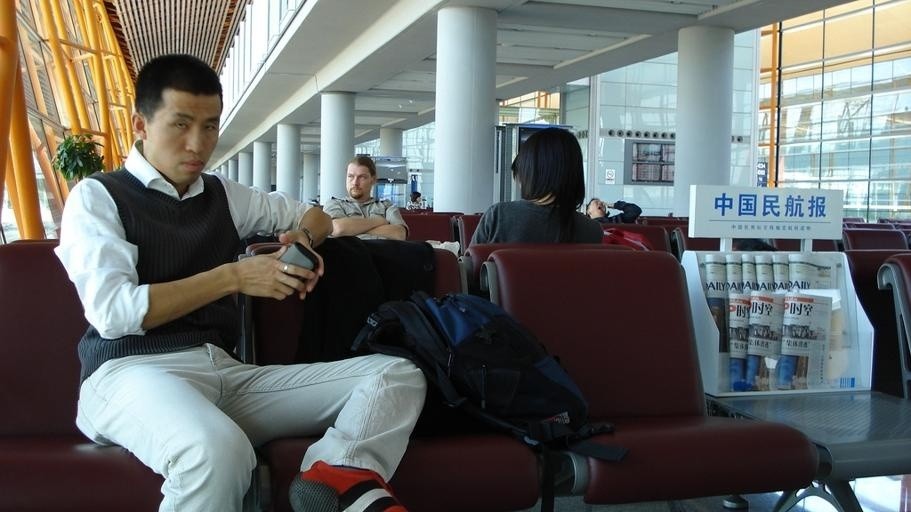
[[585, 198, 642, 225], [53, 53, 428, 512], [406, 191, 427, 210], [465, 126, 604, 245], [322, 156, 410, 242]]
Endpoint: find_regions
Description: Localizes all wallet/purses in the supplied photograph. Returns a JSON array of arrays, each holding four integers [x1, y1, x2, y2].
[[275, 242, 318, 294]]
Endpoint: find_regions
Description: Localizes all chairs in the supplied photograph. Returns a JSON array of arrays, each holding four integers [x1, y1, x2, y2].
[[1, 205, 911, 512]]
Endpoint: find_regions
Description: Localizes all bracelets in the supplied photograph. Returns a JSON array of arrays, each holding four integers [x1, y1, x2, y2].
[[300, 226, 314, 248]]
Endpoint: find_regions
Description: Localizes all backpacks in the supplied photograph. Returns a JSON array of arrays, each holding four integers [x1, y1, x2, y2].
[[600, 227, 654, 252], [350, 291, 595, 430]]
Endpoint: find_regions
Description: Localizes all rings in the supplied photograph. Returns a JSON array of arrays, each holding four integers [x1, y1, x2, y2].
[[283, 263, 288, 274]]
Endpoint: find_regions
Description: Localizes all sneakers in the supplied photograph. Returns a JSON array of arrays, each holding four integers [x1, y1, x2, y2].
[[286, 460, 409, 511]]
